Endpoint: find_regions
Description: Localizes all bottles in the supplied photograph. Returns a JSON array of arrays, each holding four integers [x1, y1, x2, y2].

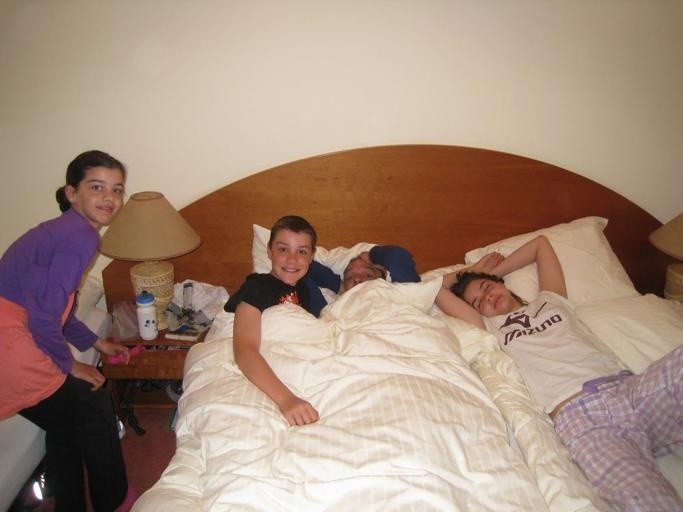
[[135, 290, 159, 341]]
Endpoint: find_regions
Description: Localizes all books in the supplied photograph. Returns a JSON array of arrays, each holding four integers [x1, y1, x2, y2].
[[164, 331, 202, 342]]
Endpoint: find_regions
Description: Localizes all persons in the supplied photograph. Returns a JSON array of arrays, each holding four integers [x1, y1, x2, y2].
[[223, 216, 321, 427], [435, 235, 683, 512], [307, 244, 422, 294], [0, 151, 132, 512]]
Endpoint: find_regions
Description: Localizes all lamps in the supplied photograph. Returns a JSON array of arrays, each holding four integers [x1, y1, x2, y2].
[[99, 191, 202, 330], [650, 212, 683, 303]]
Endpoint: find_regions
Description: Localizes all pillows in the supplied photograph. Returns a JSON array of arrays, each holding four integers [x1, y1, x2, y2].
[[75, 274, 104, 323], [465, 215, 643, 304], [252, 224, 378, 282]]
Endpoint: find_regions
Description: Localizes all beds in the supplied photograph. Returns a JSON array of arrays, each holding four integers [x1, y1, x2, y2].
[[1, 263, 111, 512], [101, 144, 682, 512]]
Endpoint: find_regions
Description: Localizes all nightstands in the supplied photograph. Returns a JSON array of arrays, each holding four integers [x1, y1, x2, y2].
[[100, 330, 208, 435]]
